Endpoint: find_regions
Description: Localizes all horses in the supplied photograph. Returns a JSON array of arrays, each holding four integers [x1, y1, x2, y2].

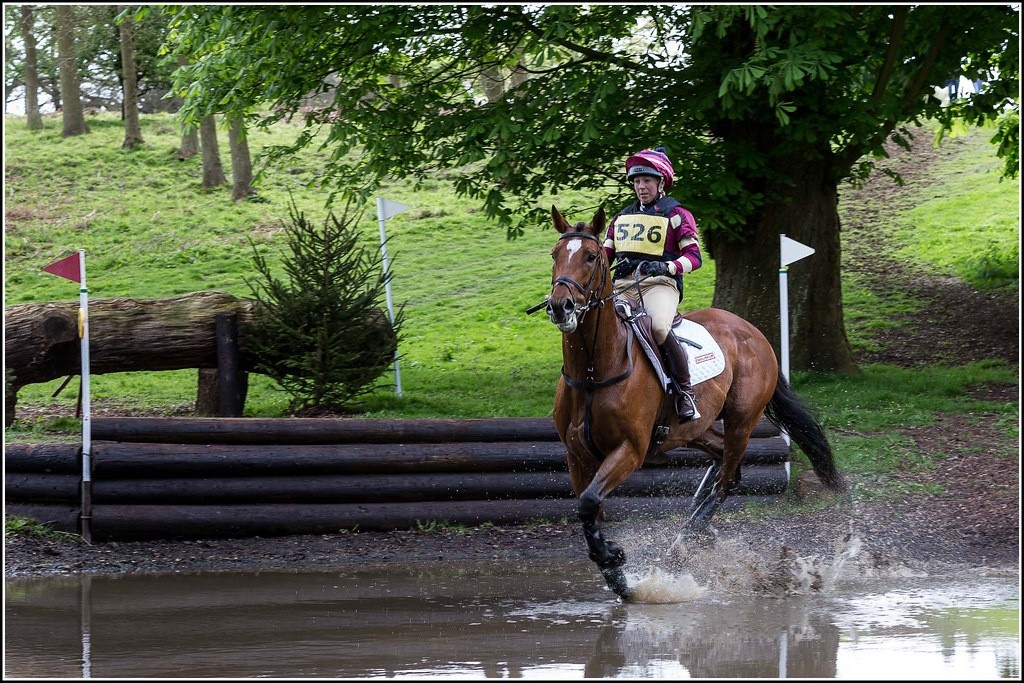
[[545, 205, 848, 600]]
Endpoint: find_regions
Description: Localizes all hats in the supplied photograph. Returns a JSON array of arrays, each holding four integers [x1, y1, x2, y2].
[[625, 147, 673, 193]]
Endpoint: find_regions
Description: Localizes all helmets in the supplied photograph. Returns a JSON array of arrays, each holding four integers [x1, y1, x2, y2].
[[628, 164, 664, 181]]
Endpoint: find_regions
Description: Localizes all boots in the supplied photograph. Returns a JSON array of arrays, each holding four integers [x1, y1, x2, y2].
[[658, 329, 696, 417]]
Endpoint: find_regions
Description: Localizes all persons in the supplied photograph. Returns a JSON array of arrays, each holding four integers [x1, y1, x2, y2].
[[604, 147, 703, 425]]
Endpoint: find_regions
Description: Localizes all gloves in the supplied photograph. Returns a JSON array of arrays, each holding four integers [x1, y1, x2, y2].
[[643, 260, 667, 277]]
